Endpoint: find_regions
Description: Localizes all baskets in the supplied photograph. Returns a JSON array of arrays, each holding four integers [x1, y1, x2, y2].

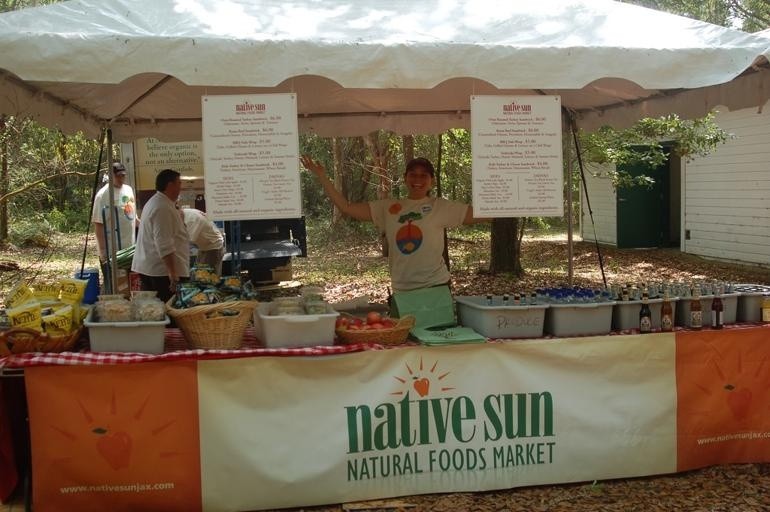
[[335, 313, 416, 346], [0, 326, 81, 353], [163, 290, 259, 351]]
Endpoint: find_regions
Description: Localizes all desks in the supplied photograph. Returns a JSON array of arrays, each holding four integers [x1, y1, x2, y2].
[[0, 322, 770, 511]]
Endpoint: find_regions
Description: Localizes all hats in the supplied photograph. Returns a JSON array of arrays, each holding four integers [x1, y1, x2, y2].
[[111, 162, 126, 171], [406, 157, 434, 177]]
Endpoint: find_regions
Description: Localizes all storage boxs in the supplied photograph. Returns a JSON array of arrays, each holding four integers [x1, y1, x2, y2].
[[737, 291, 769, 324], [84, 304, 171, 355], [543, 300, 617, 338], [612, 293, 681, 333], [676, 291, 741, 329], [453, 294, 550, 339], [253, 301, 340, 348]]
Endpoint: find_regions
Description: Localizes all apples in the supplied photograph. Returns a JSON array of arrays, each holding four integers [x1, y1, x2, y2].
[[412, 376, 429, 397], [336, 311, 397, 331]]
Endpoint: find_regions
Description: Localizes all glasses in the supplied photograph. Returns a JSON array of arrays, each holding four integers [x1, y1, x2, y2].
[[115, 173, 126, 177]]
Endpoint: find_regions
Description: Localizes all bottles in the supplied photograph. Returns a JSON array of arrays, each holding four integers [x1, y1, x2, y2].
[[760, 294, 770, 325], [711, 286, 724, 329], [687, 287, 703, 331], [486, 280, 734, 308], [660, 290, 673, 332], [639, 292, 652, 333]]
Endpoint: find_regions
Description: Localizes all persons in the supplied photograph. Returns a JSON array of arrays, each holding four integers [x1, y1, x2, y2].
[[299, 151, 494, 327], [91, 163, 140, 282], [129, 167, 193, 328], [173, 206, 226, 277]]
